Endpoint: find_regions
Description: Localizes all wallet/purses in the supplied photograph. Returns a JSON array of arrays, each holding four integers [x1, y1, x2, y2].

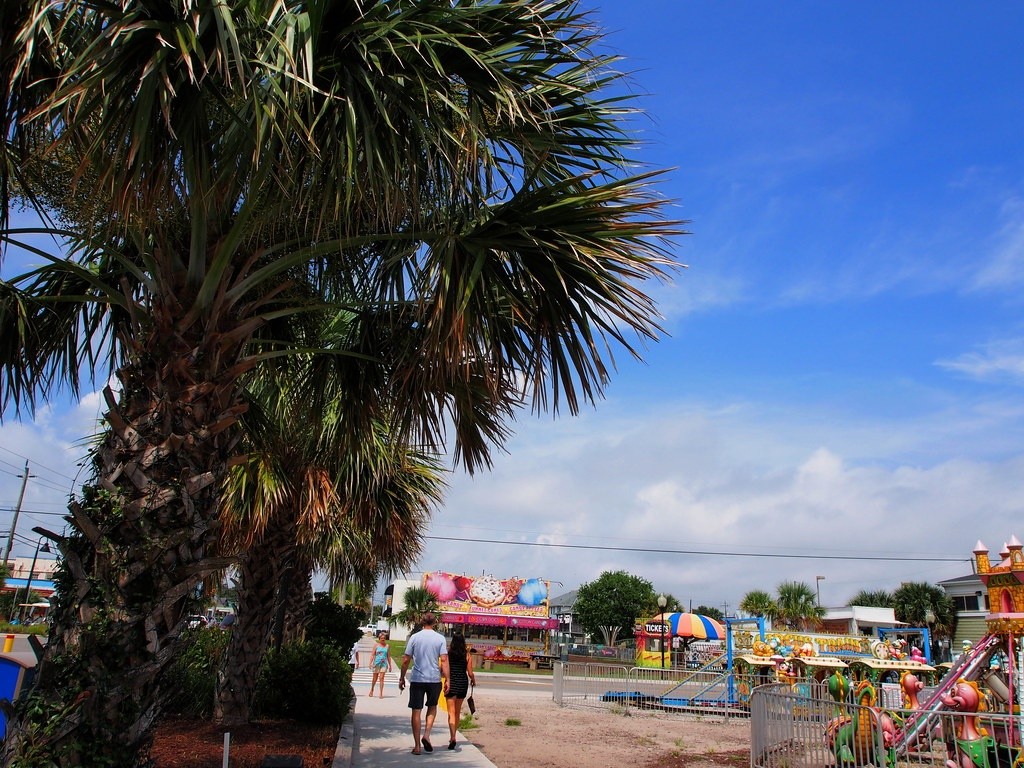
[[467, 685, 475, 715]]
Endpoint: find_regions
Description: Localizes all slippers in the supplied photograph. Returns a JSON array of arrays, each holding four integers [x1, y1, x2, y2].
[[412, 749, 421, 755], [421, 735, 433, 752]]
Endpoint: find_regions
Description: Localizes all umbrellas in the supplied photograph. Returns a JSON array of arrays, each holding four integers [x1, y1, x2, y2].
[[654, 613, 725, 651]]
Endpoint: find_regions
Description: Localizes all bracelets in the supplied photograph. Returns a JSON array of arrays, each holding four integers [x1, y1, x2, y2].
[[400, 677, 404, 681]]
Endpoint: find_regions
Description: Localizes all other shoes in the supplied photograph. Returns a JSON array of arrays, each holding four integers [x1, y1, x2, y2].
[[448, 739, 456, 749]]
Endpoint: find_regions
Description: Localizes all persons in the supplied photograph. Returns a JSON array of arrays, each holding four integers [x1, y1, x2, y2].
[[348, 642, 359, 674], [368, 632, 392, 700], [398, 613, 450, 755], [440, 634, 476, 750]]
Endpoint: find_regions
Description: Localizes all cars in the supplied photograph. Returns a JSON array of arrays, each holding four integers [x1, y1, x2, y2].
[[566, 642, 635, 659]]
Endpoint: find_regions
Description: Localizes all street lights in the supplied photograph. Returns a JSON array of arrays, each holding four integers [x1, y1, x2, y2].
[[816, 575, 826, 608], [657, 592, 668, 679], [20, 535, 50, 628]]
